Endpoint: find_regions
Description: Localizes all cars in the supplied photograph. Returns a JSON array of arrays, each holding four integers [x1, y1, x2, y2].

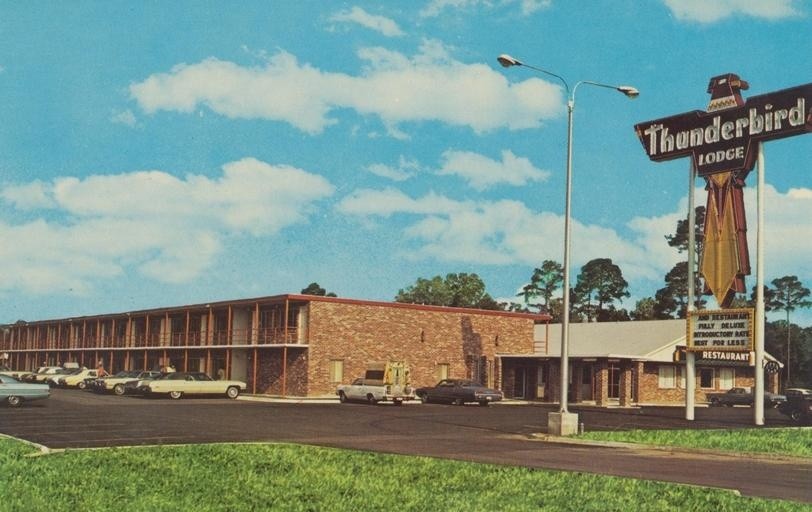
[[19, 365, 164, 395], [0, 373, 51, 407], [415, 378, 502, 407], [778, 395, 812, 420], [140, 371, 248, 400], [784, 387, 812, 400], [705, 387, 788, 409]]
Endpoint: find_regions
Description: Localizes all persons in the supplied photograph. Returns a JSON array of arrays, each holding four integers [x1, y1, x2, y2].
[[79, 364, 87, 369], [97, 365, 105, 377], [217, 367, 224, 380], [159, 364, 176, 373]]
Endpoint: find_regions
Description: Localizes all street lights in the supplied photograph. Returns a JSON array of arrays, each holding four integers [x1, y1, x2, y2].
[[497, 53, 638, 436]]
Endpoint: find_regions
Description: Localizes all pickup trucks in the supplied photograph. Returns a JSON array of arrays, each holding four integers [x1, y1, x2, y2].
[[335, 375, 416, 406]]
